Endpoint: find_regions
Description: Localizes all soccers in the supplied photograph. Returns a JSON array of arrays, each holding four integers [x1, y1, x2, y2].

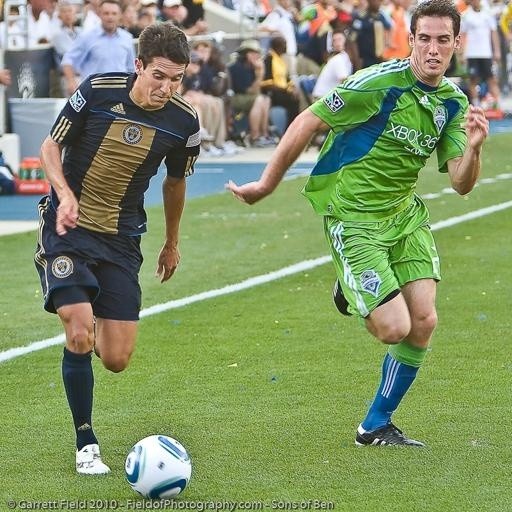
[[126, 434, 191, 500]]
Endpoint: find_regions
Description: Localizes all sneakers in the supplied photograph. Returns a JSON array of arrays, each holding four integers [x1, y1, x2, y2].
[[332, 276, 354, 318], [199, 135, 279, 156], [355, 421, 426, 448], [75, 444, 112, 475]]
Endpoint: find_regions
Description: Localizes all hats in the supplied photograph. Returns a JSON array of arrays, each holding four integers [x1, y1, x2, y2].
[[234, 39, 261, 52]]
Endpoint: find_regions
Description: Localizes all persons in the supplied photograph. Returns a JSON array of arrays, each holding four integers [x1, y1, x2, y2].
[[0, 2, 512, 158], [33, 23, 201, 475], [223, 0, 489, 448]]
